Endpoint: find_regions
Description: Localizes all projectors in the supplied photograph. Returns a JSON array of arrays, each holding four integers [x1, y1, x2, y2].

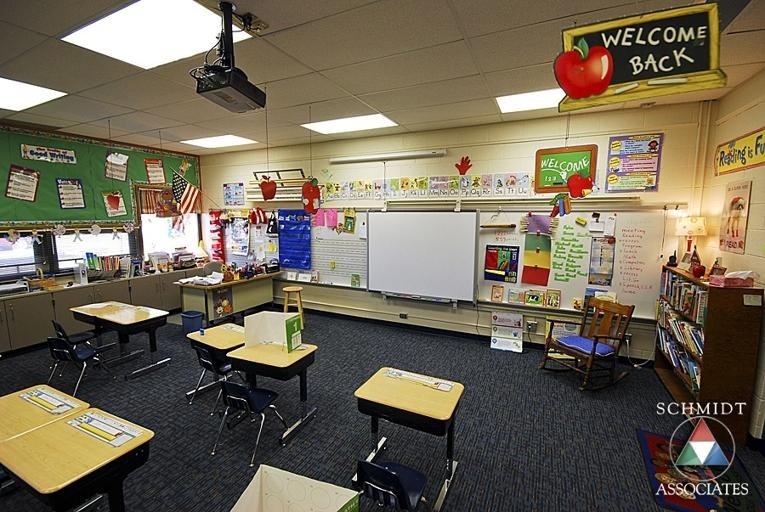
[[195, 74, 267, 114]]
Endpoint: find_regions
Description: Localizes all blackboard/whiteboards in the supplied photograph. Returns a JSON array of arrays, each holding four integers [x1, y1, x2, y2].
[[366, 208, 480, 307], [209, 205, 681, 324], [561, 3, 719, 92]]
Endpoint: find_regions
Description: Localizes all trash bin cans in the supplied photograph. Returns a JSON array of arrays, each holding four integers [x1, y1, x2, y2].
[[180, 310, 203, 334]]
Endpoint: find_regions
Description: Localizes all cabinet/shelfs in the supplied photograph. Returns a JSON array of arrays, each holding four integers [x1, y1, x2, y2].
[[0, 266, 204, 353], [656, 264, 763, 444]]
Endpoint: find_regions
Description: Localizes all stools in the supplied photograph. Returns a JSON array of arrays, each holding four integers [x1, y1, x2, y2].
[[281, 286, 308, 330]]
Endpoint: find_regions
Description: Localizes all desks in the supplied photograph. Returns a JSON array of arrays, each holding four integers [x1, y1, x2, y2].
[[0, 385, 155, 512], [186, 322, 319, 445], [352, 364, 466, 511]]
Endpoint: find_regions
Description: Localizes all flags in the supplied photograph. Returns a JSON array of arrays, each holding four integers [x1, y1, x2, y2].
[[170, 170, 199, 215]]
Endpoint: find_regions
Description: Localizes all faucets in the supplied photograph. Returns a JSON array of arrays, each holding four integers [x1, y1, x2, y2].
[[36, 268, 44, 291]]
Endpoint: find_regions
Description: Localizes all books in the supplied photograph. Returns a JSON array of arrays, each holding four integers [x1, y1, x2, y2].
[[490, 285, 505, 304], [582, 288, 608, 313], [507, 286, 562, 310], [85, 245, 208, 278], [592, 290, 617, 315], [656, 268, 708, 393]]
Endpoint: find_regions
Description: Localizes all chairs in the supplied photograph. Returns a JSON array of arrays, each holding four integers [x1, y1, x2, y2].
[[69, 300, 172, 382], [210, 379, 289, 466], [356, 458, 428, 511], [537, 294, 634, 392], [45, 319, 117, 397]]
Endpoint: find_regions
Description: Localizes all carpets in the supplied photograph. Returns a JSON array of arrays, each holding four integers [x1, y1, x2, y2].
[[636, 426, 765, 512]]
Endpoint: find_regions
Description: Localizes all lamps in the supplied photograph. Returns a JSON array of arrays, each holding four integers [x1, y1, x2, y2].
[[677, 215, 707, 251]]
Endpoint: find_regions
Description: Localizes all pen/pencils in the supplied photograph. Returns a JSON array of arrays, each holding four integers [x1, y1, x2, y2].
[[480, 224, 516, 229]]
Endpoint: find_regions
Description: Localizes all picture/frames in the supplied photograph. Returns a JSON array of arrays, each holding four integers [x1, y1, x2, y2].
[[708, 264, 727, 278]]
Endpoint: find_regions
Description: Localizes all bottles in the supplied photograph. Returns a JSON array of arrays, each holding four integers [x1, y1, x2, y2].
[[690, 245, 699, 267], [199, 327, 204, 336]]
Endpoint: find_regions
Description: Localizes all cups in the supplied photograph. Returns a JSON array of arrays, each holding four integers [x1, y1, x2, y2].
[[669, 255, 676, 263]]
